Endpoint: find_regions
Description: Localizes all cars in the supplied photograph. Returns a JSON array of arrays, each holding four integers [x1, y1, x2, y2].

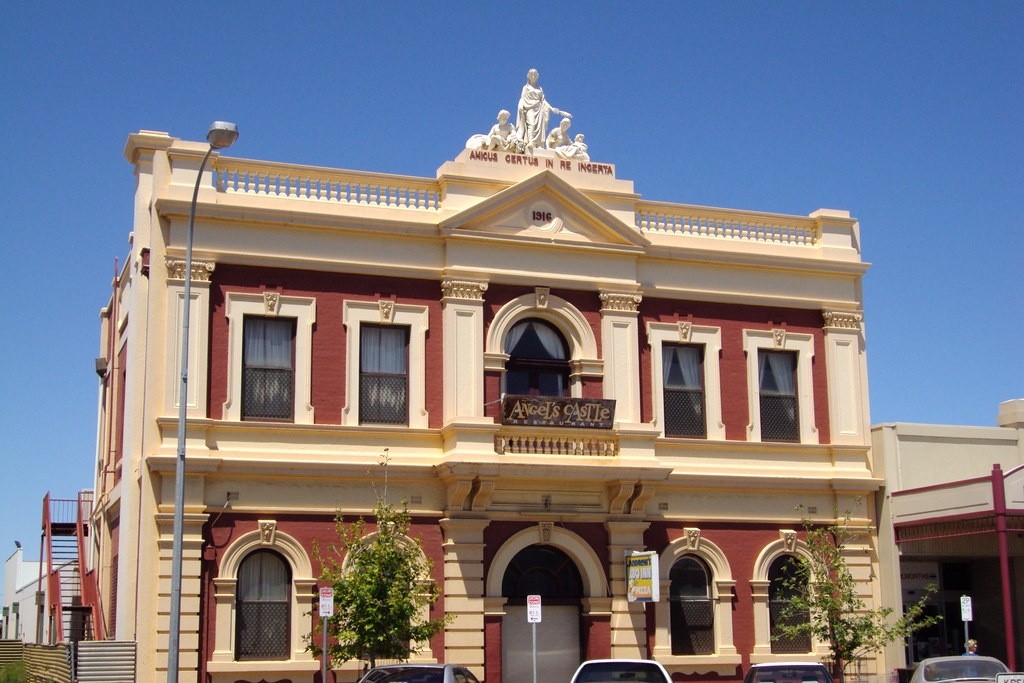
[[359, 663, 479, 683], [911, 656, 1013, 683], [745, 662, 835, 683], [570, 659, 673, 683]]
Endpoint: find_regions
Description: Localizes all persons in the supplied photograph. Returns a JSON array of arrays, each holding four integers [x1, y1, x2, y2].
[[516, 68, 573, 158], [545, 117, 582, 159], [571, 134, 591, 162], [469, 109, 518, 153], [963, 639, 980, 657]]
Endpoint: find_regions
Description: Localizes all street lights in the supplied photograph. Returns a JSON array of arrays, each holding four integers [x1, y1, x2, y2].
[[165, 120, 238, 683]]
